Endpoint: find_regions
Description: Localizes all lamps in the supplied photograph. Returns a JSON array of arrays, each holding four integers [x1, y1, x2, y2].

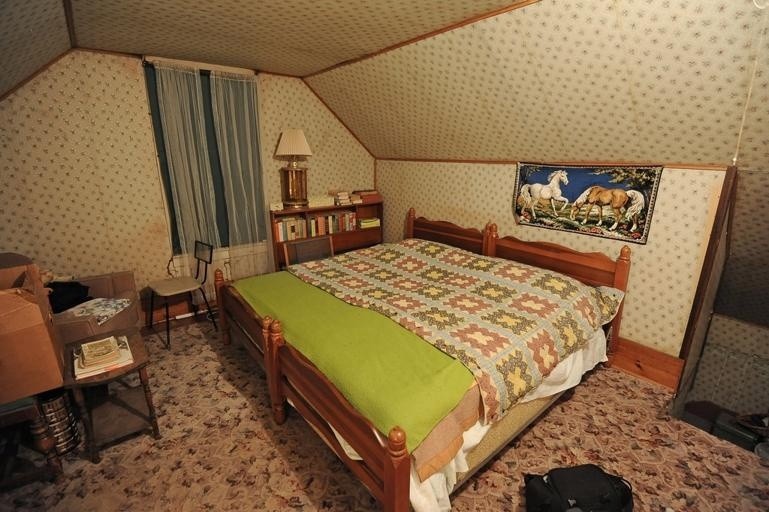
[[275, 127, 313, 211]]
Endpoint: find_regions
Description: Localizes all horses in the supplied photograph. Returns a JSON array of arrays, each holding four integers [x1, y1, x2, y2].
[[569, 183, 645, 234], [519, 169, 569, 221]]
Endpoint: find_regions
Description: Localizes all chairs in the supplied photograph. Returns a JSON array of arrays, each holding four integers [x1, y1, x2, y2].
[[148, 241, 214, 350], [283, 236, 334, 267], [45, 270, 139, 341]]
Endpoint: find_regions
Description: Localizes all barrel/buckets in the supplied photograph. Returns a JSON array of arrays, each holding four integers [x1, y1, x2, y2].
[[39, 393, 80, 456]]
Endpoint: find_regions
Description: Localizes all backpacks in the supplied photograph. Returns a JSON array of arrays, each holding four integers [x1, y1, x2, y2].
[[524, 464, 634, 512]]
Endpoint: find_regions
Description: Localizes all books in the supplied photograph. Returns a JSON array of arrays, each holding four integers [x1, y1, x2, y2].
[[273, 210, 380, 242], [327, 190, 384, 205], [73, 335, 135, 380]]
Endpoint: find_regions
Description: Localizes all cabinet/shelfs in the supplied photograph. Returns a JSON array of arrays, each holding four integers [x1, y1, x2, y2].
[[270, 201, 383, 271], [0, 396, 67, 495]]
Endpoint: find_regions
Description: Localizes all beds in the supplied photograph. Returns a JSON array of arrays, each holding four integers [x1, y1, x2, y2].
[[215, 207, 632, 512]]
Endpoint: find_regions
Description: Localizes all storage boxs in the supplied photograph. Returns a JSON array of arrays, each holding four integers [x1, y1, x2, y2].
[[1, 252, 65, 406]]
